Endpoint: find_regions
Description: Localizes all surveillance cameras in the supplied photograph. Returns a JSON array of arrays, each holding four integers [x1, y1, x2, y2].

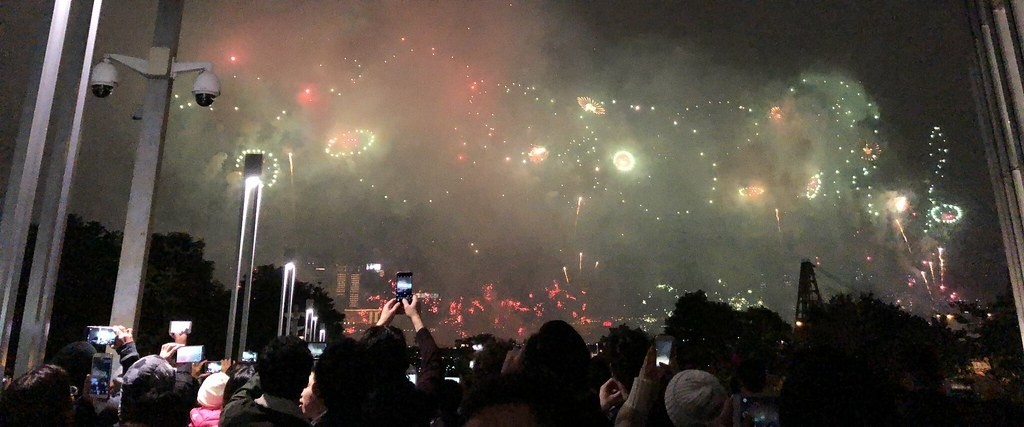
[[88, 61, 121, 97], [191, 72, 221, 109]]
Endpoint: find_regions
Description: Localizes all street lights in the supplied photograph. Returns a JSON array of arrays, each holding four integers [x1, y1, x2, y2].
[[278, 248, 296, 337], [304, 299, 327, 342], [223, 153, 265, 366]]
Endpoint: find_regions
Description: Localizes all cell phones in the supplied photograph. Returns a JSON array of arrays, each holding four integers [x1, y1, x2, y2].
[[169, 320, 193, 334], [241, 351, 259, 363], [205, 361, 223, 373], [406, 370, 417, 387], [84, 325, 118, 346], [395, 271, 413, 304], [306, 342, 328, 358], [89, 354, 113, 399], [652, 335, 674, 368], [444, 376, 460, 384], [175, 346, 203, 364]]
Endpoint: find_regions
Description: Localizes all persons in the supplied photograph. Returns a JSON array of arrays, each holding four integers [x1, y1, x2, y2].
[[0, 289, 850, 427]]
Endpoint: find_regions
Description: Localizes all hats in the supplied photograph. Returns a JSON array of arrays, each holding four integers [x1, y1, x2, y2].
[[197, 372, 230, 410], [665, 369, 732, 426]]
